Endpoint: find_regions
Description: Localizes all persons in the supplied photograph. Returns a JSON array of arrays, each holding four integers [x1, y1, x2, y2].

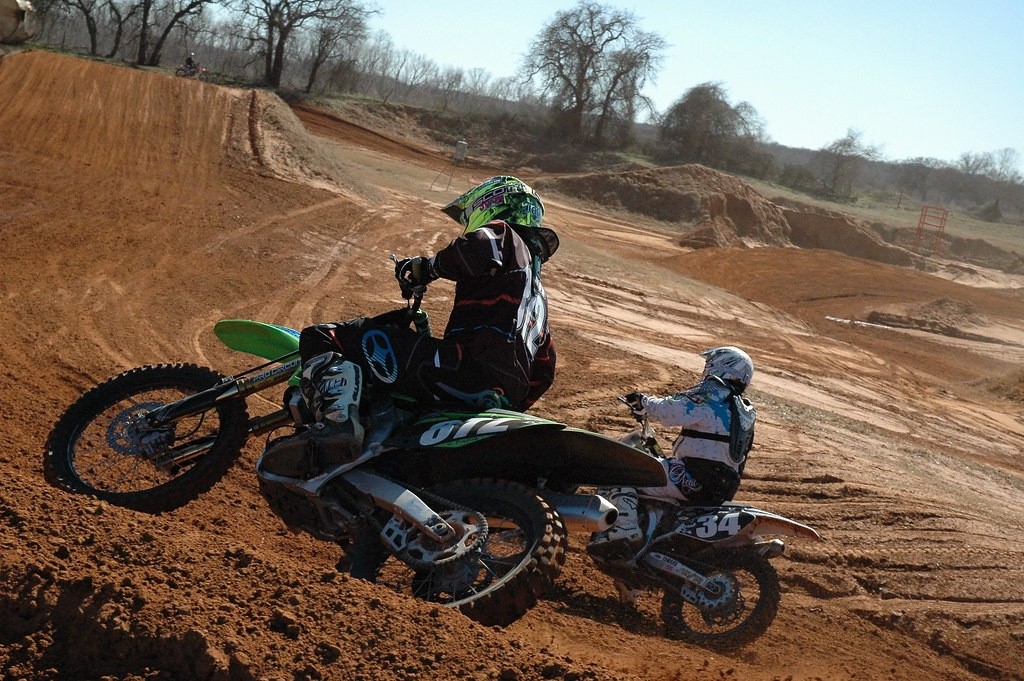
[[586, 345, 756, 554], [185, 51, 194, 78], [265, 173, 560, 463]]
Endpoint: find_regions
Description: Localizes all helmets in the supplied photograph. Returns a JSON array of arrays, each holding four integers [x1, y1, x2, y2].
[[699, 346, 753, 392], [440, 175, 545, 239]]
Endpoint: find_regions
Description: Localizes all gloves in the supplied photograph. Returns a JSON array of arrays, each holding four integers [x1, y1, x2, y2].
[[395, 256, 434, 300], [618, 393, 648, 416]]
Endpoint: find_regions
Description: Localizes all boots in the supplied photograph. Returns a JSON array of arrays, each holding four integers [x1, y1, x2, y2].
[[336, 545, 390, 583], [263, 351, 364, 476], [589, 488, 643, 549], [613, 577, 641, 607]]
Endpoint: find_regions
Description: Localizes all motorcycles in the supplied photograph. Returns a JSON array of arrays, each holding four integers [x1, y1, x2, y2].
[[590, 393, 824, 654], [175, 63, 209, 83], [40, 253, 668, 628]]
